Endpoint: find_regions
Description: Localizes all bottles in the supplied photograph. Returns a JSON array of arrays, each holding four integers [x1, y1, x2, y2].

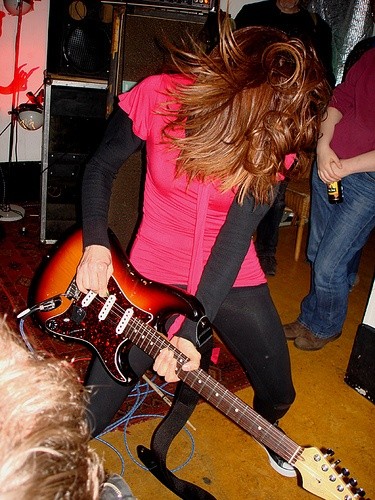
[[328, 180, 343, 203]]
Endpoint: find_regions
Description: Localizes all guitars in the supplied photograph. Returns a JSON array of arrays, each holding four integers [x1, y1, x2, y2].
[[33, 219, 367, 500]]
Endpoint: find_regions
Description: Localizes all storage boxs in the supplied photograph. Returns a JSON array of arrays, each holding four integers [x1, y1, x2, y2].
[[39, 78, 109, 244]]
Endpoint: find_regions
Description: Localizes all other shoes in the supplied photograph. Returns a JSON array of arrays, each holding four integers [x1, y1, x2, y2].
[[251, 426, 298, 477], [294, 330, 342, 351], [257, 255, 278, 276], [282, 321, 306, 340]]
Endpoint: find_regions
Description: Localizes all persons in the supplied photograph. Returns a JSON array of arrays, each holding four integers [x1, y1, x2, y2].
[[277, 44, 375, 351], [0, 315, 138, 500], [231, 0, 336, 276], [78, 20, 332, 477]]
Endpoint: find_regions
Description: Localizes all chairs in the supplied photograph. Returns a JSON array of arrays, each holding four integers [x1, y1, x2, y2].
[[285, 152, 316, 262]]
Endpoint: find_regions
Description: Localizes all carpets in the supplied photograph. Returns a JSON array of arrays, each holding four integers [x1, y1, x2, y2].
[[0, 206, 255, 432]]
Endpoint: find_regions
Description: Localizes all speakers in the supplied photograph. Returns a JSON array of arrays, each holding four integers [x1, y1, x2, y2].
[[114, 9, 207, 99], [46, 0, 115, 84]]
